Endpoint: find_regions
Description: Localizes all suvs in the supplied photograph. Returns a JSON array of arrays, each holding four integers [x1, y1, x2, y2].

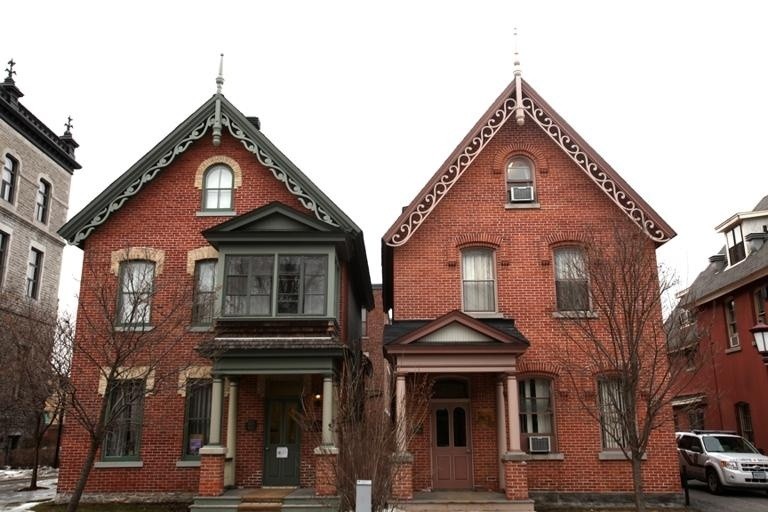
[[672, 427, 768, 495]]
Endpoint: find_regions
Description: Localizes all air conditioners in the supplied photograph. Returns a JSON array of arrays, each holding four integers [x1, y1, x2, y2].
[[728, 335, 739, 348], [529, 435, 549, 453], [510, 186, 534, 201]]
